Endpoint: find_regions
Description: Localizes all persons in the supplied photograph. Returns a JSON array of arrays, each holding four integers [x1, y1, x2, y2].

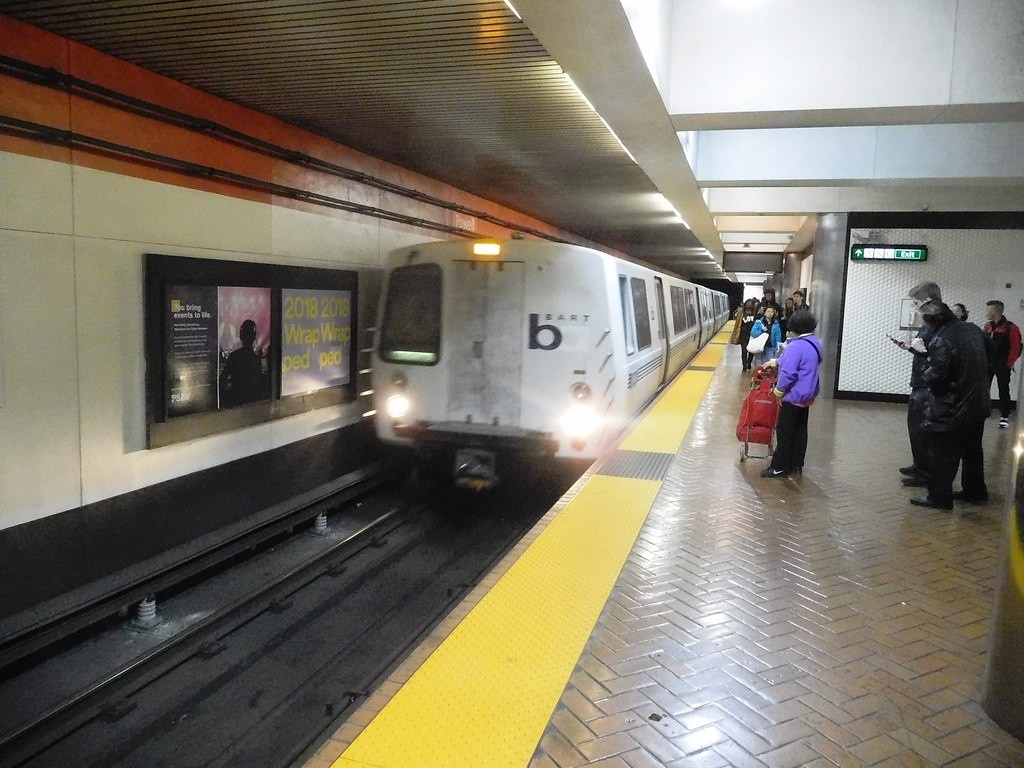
[[884, 280, 1024, 743], [730, 288, 810, 373], [219, 319, 269, 400], [760, 309, 823, 478]]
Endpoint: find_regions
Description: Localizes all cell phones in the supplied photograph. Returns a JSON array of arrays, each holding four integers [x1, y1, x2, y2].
[[887, 334, 904, 343]]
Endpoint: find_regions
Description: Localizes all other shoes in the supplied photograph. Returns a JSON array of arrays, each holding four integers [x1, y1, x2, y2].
[[1000, 418, 1008, 429], [953, 490, 988, 504], [900, 464, 919, 477], [760, 466, 789, 478], [748, 361, 752, 370], [742, 366, 747, 373], [900, 478, 928, 489], [910, 496, 953, 511], [791, 465, 802, 469]]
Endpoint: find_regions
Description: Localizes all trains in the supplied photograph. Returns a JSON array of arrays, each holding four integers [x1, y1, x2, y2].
[[369, 240, 729, 508]]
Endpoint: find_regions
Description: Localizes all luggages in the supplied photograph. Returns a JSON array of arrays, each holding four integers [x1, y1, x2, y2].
[[736, 365, 781, 461]]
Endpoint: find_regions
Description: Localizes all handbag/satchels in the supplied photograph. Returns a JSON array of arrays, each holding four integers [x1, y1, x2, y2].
[[746, 321, 769, 353]]
